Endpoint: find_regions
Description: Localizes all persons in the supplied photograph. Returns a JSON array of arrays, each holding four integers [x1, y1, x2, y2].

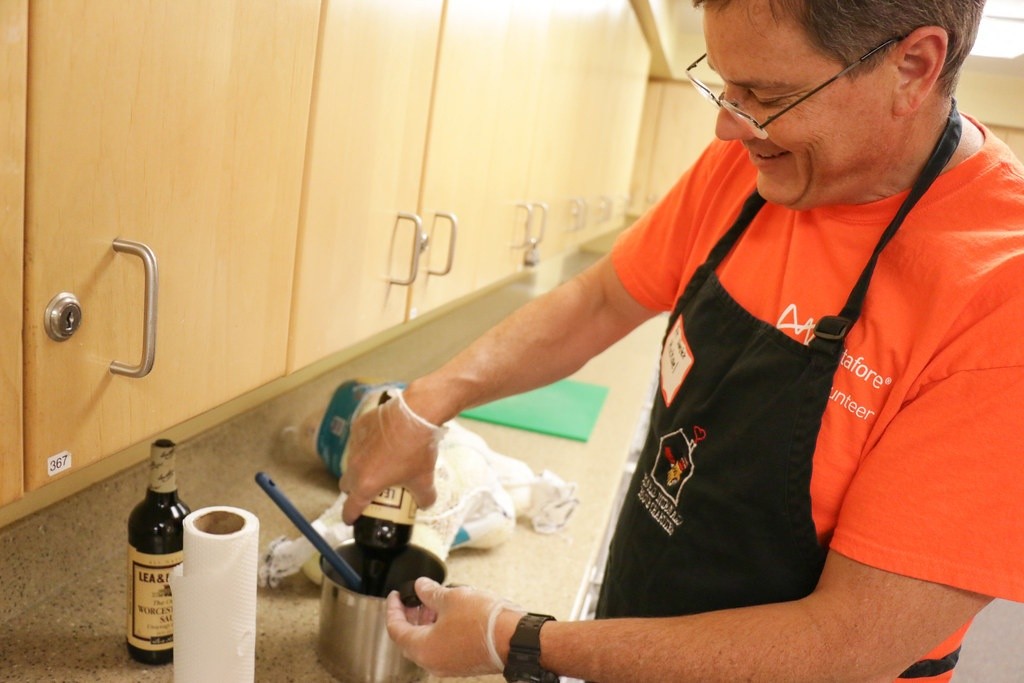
[[339, 0, 1024, 683]]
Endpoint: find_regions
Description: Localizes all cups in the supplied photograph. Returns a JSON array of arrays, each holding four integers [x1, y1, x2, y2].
[[319, 537, 450, 683]]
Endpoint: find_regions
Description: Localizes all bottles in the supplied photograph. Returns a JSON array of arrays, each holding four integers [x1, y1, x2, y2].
[[126, 439, 192, 664], [352, 485, 417, 598]]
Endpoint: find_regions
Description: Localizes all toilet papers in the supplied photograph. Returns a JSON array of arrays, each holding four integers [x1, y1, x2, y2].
[[170, 505, 260, 683]]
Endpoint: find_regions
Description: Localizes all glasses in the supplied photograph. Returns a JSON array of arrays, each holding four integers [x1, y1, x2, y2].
[[685, 35, 905, 140]]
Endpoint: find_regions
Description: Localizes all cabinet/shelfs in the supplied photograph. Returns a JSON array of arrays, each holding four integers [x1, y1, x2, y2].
[[0, 0, 653, 530]]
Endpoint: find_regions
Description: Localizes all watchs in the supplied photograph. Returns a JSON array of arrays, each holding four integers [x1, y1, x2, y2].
[[503, 611, 561, 683]]
